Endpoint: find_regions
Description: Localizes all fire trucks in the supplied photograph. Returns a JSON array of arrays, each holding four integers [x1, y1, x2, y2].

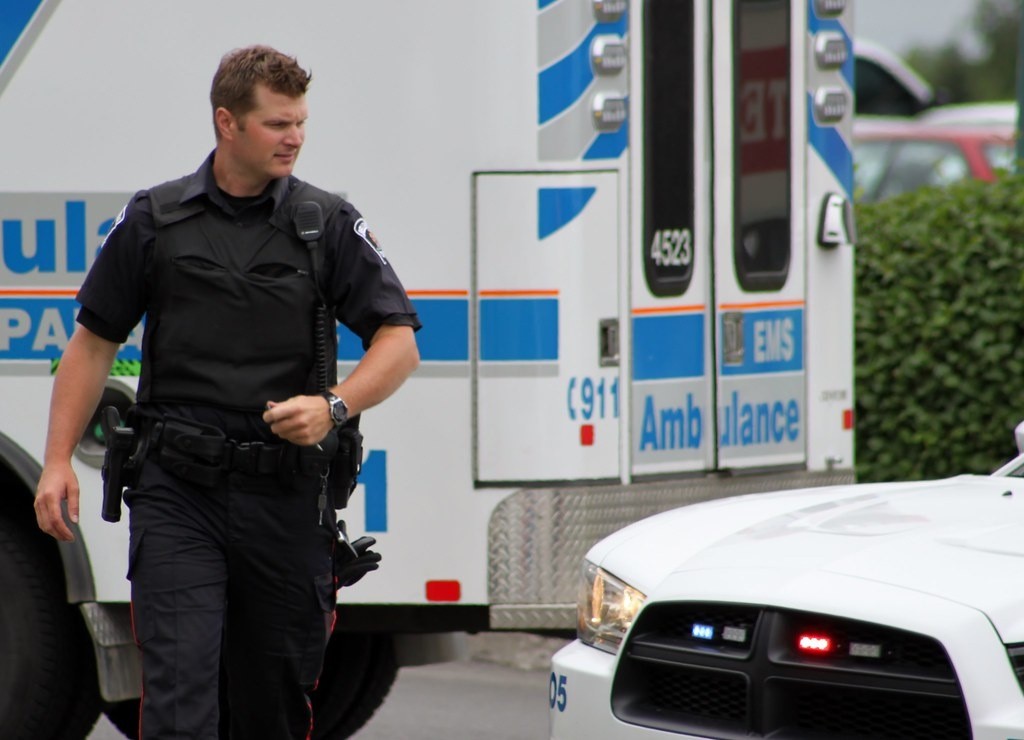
[[0, 0, 854, 740]]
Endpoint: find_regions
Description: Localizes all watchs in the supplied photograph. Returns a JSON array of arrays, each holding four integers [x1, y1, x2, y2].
[[321, 391, 348, 429]]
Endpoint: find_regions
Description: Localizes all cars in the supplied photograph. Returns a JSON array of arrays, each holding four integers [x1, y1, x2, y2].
[[856, 124, 1018, 199], [551, 474, 1024, 740]]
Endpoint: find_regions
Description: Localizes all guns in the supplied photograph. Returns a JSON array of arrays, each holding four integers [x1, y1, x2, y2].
[[98, 403, 135, 524]]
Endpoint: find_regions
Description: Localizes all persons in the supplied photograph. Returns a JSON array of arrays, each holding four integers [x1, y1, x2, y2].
[[34, 47, 422, 740]]
